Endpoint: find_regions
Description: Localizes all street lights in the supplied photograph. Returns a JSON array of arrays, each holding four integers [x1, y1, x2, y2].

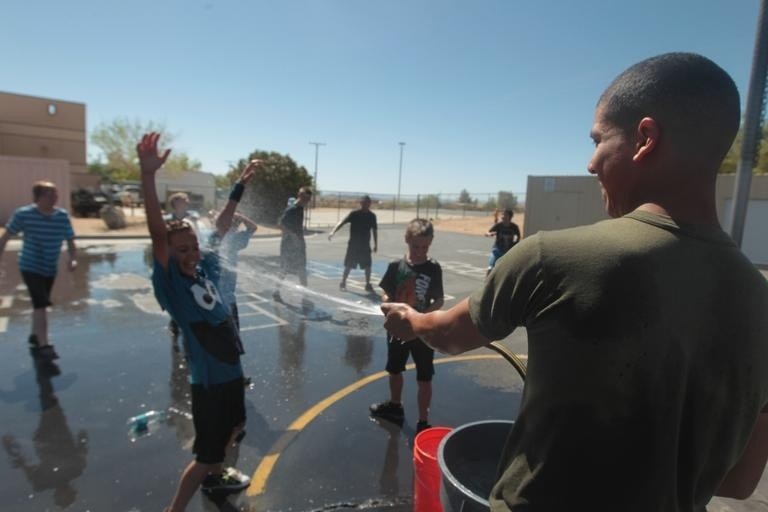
[[398, 142, 406, 209], [308, 142, 326, 207]]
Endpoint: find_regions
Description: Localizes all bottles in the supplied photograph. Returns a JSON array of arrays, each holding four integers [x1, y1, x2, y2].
[[126, 409, 165, 431]]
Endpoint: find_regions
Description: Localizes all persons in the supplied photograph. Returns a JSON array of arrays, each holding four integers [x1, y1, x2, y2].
[[484, 210, 521, 272], [273, 310, 311, 394], [159, 339, 199, 460], [382, 47, 768, 511], [369, 218, 445, 431], [0, 180, 78, 359], [136, 130, 264, 512], [163, 192, 205, 335], [342, 332, 375, 369], [206, 207, 259, 386], [327, 194, 378, 294], [274, 186, 327, 309], [0, 358, 90, 509], [372, 417, 419, 511]]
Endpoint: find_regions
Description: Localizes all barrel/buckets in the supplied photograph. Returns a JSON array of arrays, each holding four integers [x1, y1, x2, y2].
[[438, 420, 515, 512], [413, 427, 453, 512]]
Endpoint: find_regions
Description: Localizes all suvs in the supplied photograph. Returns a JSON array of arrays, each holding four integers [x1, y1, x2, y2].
[[72, 185, 142, 218]]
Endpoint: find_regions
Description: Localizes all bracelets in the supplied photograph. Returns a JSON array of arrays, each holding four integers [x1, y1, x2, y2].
[[228, 183, 247, 204]]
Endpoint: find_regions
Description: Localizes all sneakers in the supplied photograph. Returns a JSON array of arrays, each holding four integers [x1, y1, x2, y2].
[[339, 282, 347, 292], [365, 283, 374, 292], [369, 399, 406, 417], [272, 289, 314, 306], [199, 466, 251, 491], [28, 334, 60, 360], [416, 419, 432, 433]]
